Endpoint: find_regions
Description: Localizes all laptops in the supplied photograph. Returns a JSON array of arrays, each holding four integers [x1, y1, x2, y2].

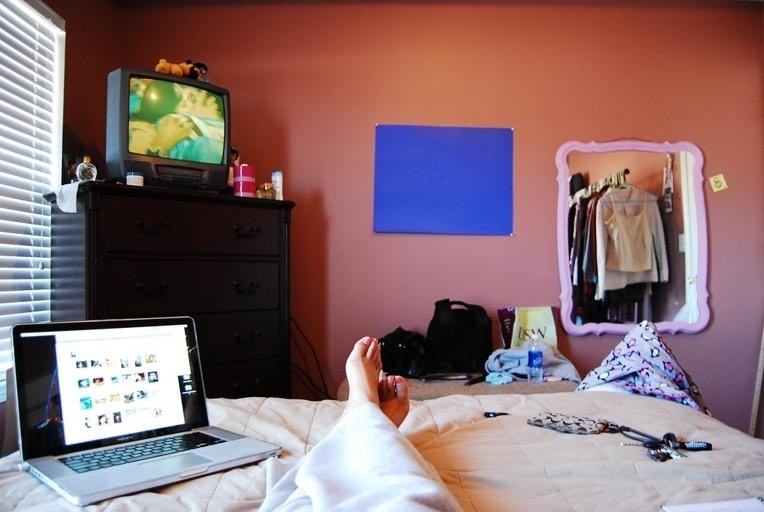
[[10, 314, 284, 507]]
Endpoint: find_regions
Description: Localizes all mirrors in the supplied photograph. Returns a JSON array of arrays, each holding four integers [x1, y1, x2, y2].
[[553, 137, 712, 338]]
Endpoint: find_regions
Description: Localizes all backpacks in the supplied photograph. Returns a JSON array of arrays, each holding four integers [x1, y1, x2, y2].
[[424, 298, 495, 375]]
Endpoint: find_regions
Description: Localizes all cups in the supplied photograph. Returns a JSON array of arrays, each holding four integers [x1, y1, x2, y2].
[[126, 170, 143, 187]]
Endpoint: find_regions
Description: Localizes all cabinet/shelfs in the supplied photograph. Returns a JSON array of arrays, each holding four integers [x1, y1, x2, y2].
[[42, 178, 296, 399]]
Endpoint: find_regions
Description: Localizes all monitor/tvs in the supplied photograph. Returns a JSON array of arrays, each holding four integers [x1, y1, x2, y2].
[[105, 67, 231, 194]]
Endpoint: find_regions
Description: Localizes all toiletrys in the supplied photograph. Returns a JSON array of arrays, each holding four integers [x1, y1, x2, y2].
[[74, 157, 96, 182], [270, 168, 283, 202], [255, 181, 274, 200], [124, 171, 144, 188]]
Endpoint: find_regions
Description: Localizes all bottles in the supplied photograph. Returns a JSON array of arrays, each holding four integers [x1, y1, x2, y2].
[[271, 169, 283, 200], [527, 334, 545, 385], [77, 154, 99, 181]]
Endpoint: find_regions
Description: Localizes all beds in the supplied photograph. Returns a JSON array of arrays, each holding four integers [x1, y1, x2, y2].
[[0, 391, 764, 512]]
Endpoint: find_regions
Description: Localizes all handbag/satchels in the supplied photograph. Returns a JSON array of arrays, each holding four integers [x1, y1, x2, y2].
[[496, 306, 559, 358], [375, 326, 429, 379]]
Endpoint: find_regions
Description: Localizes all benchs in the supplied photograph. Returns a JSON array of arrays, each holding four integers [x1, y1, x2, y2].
[[336, 370, 586, 401]]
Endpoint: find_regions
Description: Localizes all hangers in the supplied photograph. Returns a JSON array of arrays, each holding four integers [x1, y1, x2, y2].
[[565, 170, 660, 205]]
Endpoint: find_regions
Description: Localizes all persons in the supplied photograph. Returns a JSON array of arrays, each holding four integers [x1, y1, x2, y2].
[[250, 336, 464, 512], [129, 78, 224, 161]]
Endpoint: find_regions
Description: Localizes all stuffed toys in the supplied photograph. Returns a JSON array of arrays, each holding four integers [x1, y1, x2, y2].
[[153, 57, 206, 78]]
